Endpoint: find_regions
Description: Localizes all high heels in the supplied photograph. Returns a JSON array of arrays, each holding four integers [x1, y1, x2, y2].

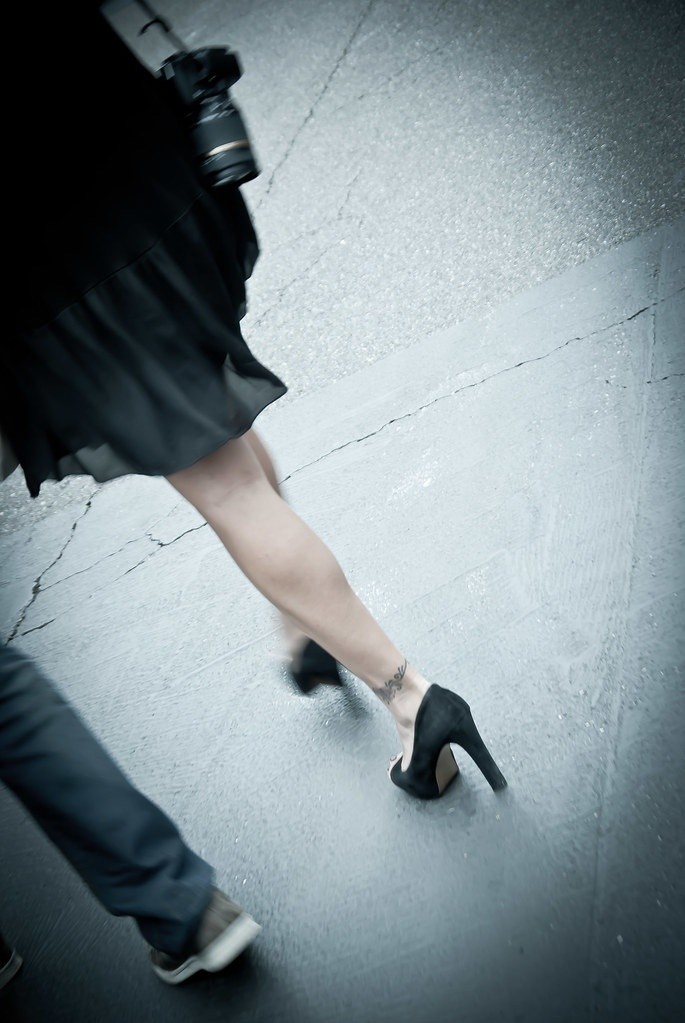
[[389, 686, 506, 800], [291, 640, 347, 697]]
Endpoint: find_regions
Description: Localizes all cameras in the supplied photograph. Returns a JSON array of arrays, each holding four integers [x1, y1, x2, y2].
[[145, 41, 258, 194]]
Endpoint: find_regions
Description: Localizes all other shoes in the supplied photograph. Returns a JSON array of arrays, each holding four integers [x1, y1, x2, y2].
[[147, 884, 262, 983], [0, 933, 24, 989]]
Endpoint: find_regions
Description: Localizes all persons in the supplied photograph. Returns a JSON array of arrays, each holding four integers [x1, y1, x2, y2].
[[0, 0, 510, 799], [0, 644, 261, 988]]
